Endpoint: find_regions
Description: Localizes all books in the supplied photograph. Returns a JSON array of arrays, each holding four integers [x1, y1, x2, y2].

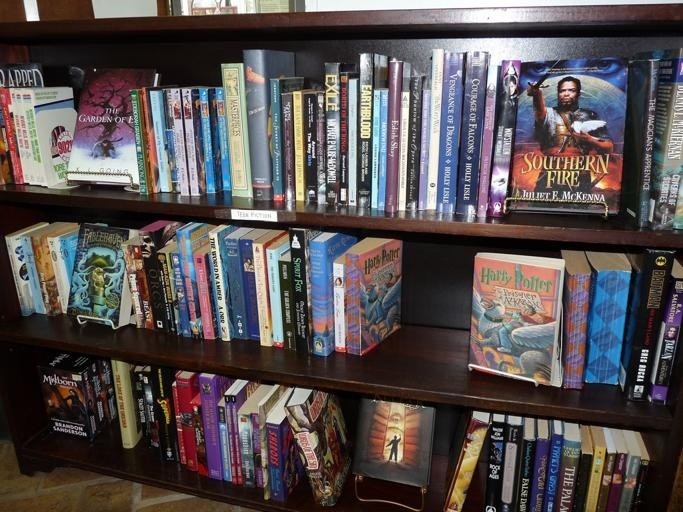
[[469, 247, 681, 401], [3, 43, 682, 211], [2, 221, 403, 356], [38, 342, 349, 504], [446, 410, 650, 512]]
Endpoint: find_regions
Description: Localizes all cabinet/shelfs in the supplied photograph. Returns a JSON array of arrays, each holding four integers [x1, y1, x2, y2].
[[3, 1, 680, 510]]
